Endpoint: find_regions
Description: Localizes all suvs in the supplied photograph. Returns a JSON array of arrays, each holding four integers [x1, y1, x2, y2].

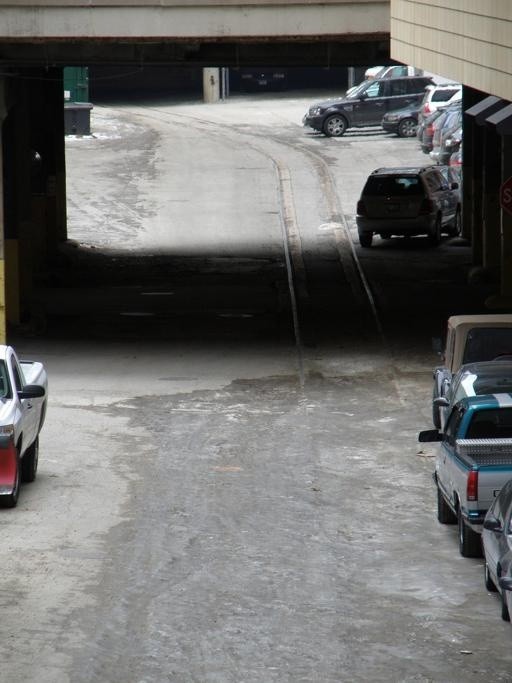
[[356, 164, 462, 250]]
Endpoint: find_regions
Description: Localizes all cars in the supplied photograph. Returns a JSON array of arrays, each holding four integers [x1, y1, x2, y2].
[[240, 71, 288, 91], [414, 311, 512, 628], [301, 63, 463, 195]]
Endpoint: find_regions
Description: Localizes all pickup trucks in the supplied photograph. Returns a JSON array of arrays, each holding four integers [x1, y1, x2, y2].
[[0, 341, 48, 507]]
